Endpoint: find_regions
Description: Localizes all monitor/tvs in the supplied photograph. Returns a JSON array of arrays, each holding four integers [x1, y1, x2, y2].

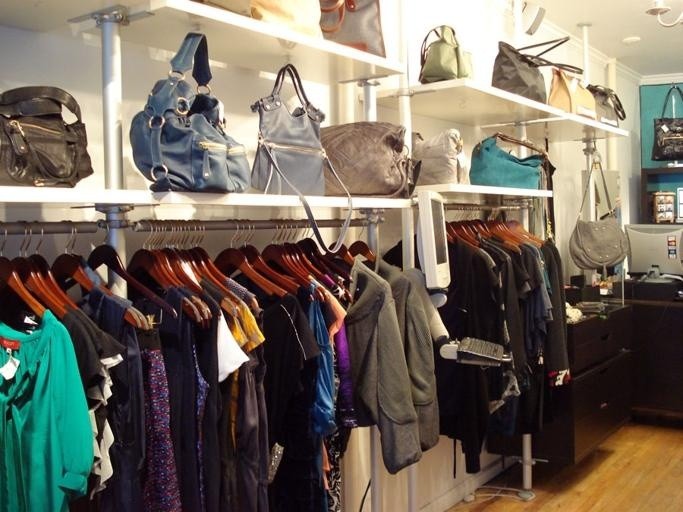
[[624, 223, 683, 284]]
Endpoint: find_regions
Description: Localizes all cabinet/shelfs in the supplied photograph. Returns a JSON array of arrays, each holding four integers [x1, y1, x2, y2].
[[488, 303, 631, 463], [641, 167, 683, 225], [358, 78, 629, 197], [0, 0, 412, 208]]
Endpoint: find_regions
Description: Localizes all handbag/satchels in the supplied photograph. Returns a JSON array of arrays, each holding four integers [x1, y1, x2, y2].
[[419, 23, 471, 82], [0, 85, 95, 186], [412, 128, 464, 186], [651, 87, 683, 160], [322, 122, 424, 197], [569, 202, 629, 270], [252, 64, 328, 196], [469, 131, 551, 190], [495, 37, 549, 103], [587, 83, 625, 127], [130, 33, 252, 193], [549, 67, 596, 120], [206, 1, 384, 59]]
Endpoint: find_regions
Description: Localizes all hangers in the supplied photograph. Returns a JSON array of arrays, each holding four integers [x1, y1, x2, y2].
[[0, 218, 376, 331], [444, 204, 544, 249]]
